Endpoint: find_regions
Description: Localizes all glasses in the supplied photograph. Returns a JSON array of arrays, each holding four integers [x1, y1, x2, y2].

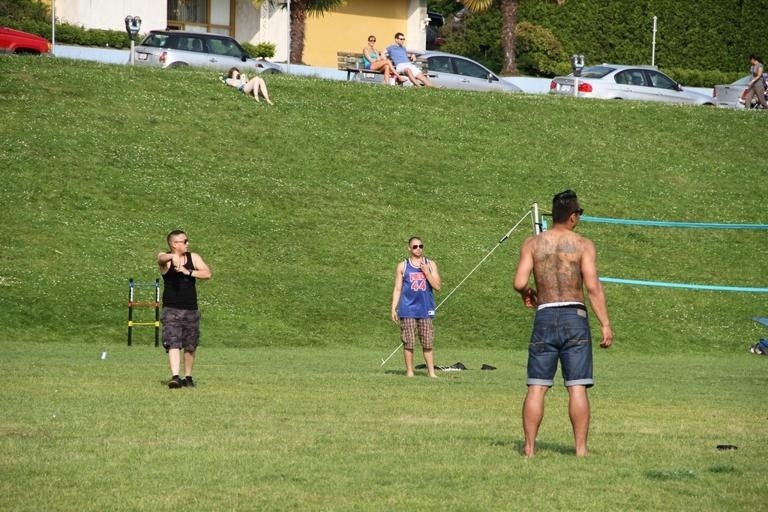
[[174, 240, 188, 244], [370, 40, 376, 42], [398, 38, 405, 40], [413, 245, 423, 249], [572, 209, 584, 215]]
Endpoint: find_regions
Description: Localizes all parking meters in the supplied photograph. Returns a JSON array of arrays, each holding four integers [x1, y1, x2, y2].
[[570, 53, 585, 97], [124, 15, 142, 69]]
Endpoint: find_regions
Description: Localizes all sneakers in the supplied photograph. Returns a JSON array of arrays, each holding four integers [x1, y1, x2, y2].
[[168, 378, 193, 388]]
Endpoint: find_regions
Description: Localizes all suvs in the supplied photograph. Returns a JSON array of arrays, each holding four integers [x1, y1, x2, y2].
[[130, 31, 285, 81]]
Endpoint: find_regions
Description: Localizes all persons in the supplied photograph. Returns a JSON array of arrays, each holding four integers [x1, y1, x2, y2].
[[380, 31, 446, 90], [223, 67, 276, 106], [157, 229, 212, 390], [363, 35, 408, 88], [740, 53, 768, 109], [390, 236, 440, 378], [512, 190, 613, 459]]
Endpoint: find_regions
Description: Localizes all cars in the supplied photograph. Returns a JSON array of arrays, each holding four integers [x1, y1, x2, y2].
[[359, 49, 525, 97], [549, 62, 719, 111], [713, 70, 768, 111], [0, 25, 53, 57]]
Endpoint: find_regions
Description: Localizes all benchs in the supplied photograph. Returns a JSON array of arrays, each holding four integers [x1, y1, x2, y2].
[[337, 52, 437, 89]]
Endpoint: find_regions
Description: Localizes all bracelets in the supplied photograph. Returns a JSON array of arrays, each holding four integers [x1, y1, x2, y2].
[[189, 269, 193, 276], [751, 80, 754, 82]]
